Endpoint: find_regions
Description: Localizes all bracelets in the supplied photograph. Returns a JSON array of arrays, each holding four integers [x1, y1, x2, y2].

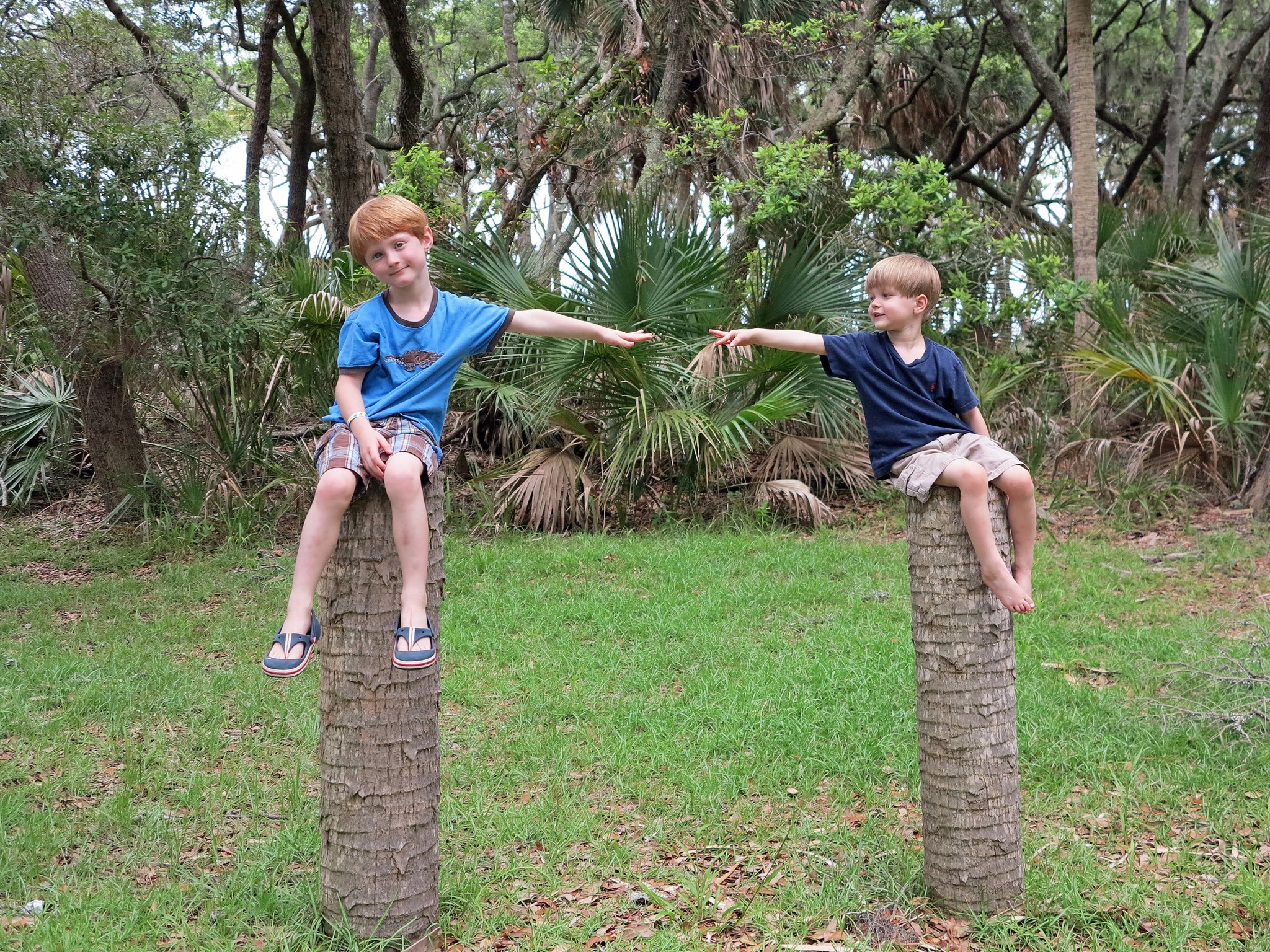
[[347, 411, 369, 429]]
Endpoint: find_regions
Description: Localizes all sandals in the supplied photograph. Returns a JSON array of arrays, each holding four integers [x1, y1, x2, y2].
[[260, 608, 321, 678], [392, 611, 438, 669]]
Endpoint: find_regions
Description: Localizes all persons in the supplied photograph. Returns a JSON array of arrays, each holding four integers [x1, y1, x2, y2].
[[261, 194, 656, 677], [707, 255, 1038, 614]]
